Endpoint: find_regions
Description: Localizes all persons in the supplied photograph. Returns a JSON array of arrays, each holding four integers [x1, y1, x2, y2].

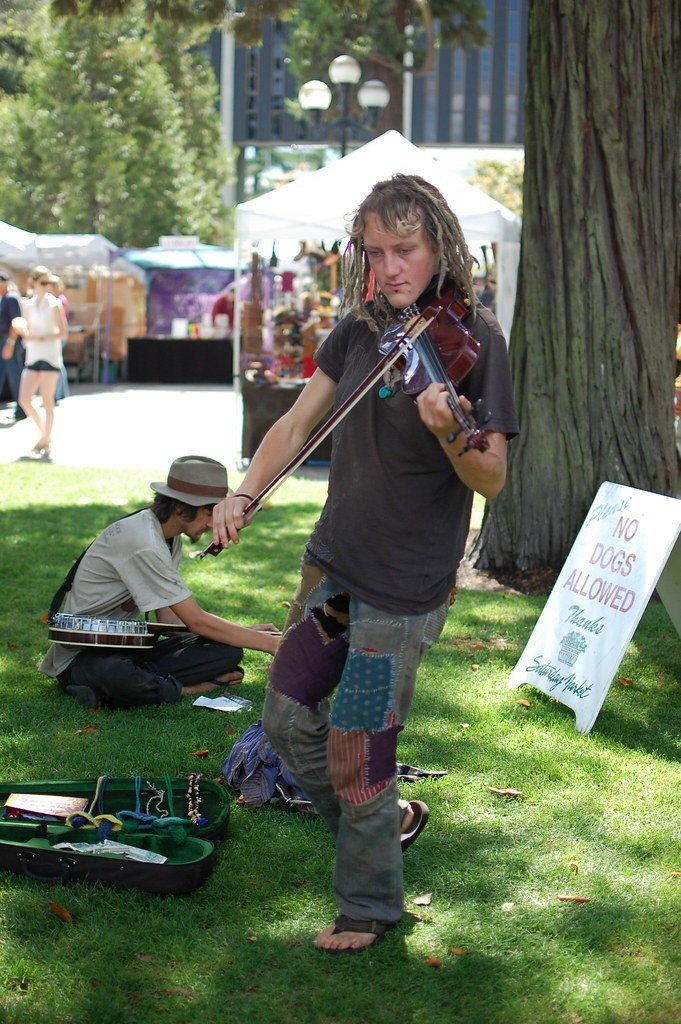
[[39, 454, 282, 710], [19, 264, 68, 455], [211, 286, 234, 330], [210, 175, 520, 955], [0, 274, 70, 421]]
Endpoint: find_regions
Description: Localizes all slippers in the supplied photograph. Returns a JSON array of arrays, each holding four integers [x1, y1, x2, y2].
[[397, 800, 432, 852], [313, 915, 396, 954], [205, 666, 246, 688]]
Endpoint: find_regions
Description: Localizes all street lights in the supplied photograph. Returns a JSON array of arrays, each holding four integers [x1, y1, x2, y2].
[[295, 51, 390, 160]]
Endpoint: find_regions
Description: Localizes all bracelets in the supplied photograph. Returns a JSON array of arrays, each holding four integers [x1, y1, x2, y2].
[[232, 493, 253, 503]]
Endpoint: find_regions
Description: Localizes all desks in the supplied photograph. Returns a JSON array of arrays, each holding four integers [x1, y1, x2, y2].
[[125, 336, 234, 384], [240, 378, 331, 463]]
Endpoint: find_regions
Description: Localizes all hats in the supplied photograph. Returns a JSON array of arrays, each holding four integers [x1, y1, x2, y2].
[[149, 455, 236, 506]]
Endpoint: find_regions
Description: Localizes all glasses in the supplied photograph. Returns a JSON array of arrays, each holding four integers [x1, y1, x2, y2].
[[36, 280, 49, 286]]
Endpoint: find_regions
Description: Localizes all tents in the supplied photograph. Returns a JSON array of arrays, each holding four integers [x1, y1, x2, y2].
[[234, 128, 523, 471]]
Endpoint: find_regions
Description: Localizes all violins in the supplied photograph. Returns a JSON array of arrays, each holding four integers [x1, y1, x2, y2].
[[376, 277, 495, 458]]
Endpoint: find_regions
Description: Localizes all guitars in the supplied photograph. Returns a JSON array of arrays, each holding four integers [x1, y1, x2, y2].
[[46, 611, 285, 651]]
